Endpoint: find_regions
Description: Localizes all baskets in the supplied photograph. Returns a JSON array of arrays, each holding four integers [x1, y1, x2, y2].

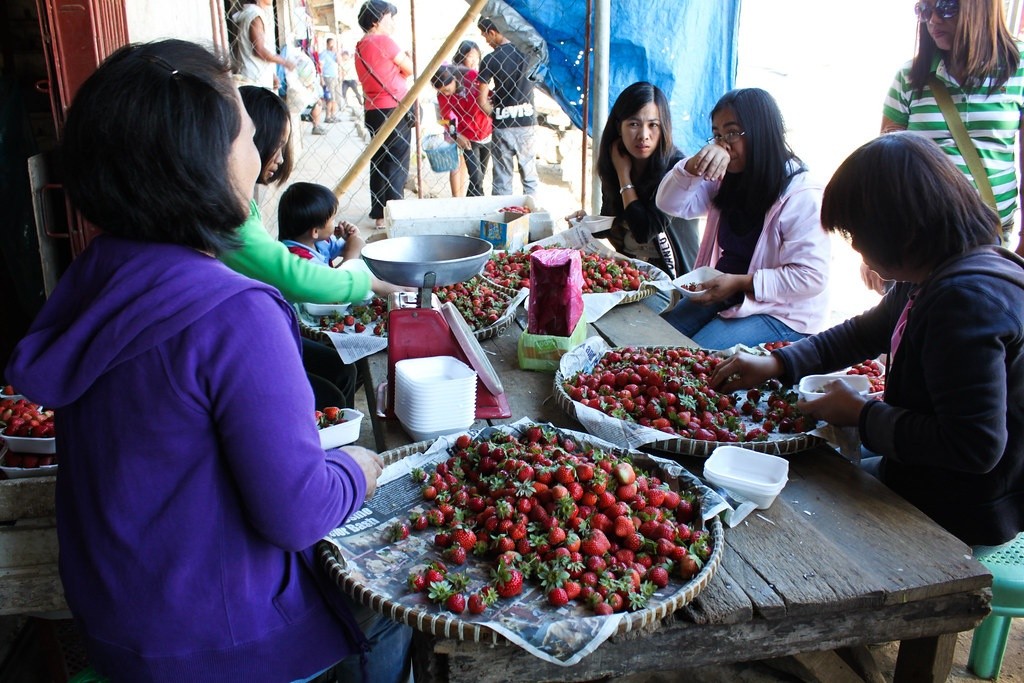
[[421, 134, 459, 173]]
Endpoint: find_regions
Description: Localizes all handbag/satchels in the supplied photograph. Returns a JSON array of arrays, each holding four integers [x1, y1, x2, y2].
[[405, 100, 423, 128]]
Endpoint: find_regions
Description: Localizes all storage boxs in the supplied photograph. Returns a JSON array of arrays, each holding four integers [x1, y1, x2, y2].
[[517, 314, 586, 371], [382, 192, 552, 241], [477, 213, 531, 252]]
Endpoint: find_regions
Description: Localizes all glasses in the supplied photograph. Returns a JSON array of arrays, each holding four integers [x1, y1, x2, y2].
[[914, 0, 959, 24], [706, 131, 746, 145]]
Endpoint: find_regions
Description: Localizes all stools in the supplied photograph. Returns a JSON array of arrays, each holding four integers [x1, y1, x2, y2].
[[966, 531, 1024, 680]]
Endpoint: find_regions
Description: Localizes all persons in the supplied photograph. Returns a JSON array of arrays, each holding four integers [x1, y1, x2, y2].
[[231, 0, 296, 97], [565, 81, 701, 277], [656, 87, 826, 352], [218, 83, 418, 408], [318, 39, 342, 124], [9, 39, 415, 683], [878, 0, 1024, 260], [355, 0, 414, 227], [477, 16, 542, 197], [336, 49, 364, 108], [277, 180, 366, 388], [301, 41, 328, 137], [431, 63, 492, 196], [446, 41, 482, 196], [709, 131, 1024, 544]]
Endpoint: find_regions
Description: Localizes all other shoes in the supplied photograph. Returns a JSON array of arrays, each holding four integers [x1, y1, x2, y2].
[[325, 115, 342, 123], [312, 126, 328, 136]]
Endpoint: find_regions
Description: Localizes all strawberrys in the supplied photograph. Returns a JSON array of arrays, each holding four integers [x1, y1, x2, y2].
[[314, 406, 348, 429], [319, 298, 387, 335], [577, 249, 650, 294], [388, 423, 713, 619], [481, 242, 575, 287], [563, 345, 822, 442], [763, 339, 886, 394], [498, 205, 531, 214], [434, 276, 506, 331], [681, 282, 697, 291], [0, 384, 57, 467]]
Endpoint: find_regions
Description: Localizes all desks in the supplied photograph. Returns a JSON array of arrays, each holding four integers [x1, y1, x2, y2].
[[359, 306, 993, 683]]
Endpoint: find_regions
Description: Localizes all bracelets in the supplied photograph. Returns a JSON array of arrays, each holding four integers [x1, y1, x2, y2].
[[619, 184, 634, 193]]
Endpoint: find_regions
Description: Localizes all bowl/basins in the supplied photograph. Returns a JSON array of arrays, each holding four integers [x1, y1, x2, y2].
[[569, 214, 616, 234], [671, 266, 724, 298], [316, 408, 364, 450], [701, 445, 790, 511], [0, 386, 58, 478], [393, 355, 479, 442]]
